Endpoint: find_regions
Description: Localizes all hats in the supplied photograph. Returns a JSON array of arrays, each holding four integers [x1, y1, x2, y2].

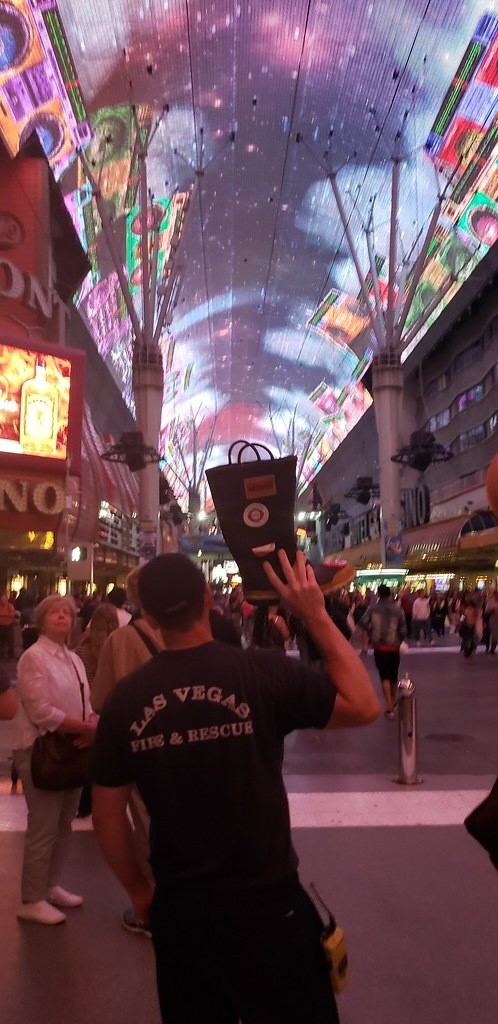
[[137, 552, 202, 619], [108, 588, 126, 607]]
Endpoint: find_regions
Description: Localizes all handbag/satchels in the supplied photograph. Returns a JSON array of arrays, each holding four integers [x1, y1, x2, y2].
[[29, 730, 95, 791], [205, 439, 298, 592]]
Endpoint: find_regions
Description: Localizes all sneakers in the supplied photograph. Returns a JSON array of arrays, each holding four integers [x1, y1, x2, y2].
[[121, 908, 152, 938], [16, 898, 67, 926], [44, 885, 83, 908]]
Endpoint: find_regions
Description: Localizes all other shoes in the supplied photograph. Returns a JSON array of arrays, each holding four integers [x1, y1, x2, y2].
[[385, 712, 395, 720], [394, 703, 399, 711]]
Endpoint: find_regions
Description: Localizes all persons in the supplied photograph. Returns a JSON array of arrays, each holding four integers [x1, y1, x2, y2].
[[92, 548, 383, 1024], [0, 566, 498, 1024]]
